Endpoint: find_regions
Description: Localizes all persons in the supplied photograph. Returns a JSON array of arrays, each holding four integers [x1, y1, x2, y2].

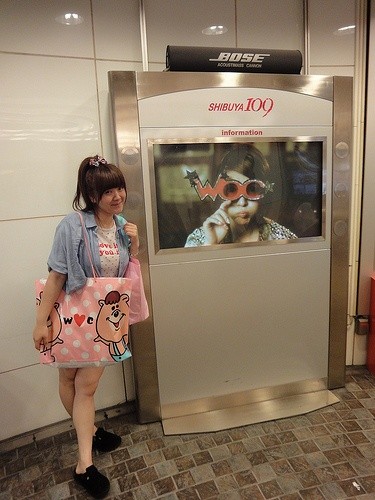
[[183, 143, 298, 247], [31, 153, 142, 500]]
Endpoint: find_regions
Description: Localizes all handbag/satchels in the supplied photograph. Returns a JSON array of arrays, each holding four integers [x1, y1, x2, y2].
[[123, 256, 150, 326], [35, 212, 132, 368]]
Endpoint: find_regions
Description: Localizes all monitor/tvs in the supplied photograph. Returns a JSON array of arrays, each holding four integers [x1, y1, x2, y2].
[[147, 133, 328, 251]]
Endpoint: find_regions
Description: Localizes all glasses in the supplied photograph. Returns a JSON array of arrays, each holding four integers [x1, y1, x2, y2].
[[184, 170, 275, 202]]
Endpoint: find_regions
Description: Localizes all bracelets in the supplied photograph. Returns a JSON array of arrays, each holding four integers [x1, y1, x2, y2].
[[130, 249, 139, 258]]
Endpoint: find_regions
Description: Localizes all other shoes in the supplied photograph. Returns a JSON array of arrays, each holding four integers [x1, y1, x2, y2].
[[92, 427, 121, 452], [73, 464, 110, 499]]
